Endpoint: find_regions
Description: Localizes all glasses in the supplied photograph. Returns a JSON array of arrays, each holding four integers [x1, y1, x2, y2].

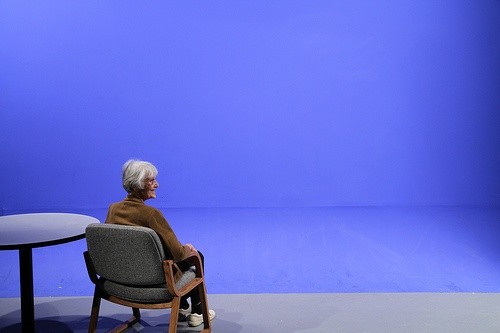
[[143, 177, 158, 186]]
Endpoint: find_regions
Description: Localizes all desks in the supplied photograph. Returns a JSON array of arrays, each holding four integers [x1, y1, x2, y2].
[[0, 212, 101, 333]]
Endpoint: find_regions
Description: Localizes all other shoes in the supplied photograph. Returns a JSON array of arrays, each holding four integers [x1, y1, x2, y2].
[[177, 306, 192, 323], [186, 309, 216, 327]]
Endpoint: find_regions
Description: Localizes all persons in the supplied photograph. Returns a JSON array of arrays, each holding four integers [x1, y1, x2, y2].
[[104, 158, 216, 327]]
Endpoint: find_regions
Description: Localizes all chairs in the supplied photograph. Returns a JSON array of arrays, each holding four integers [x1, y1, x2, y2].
[[83, 223, 212, 333]]
